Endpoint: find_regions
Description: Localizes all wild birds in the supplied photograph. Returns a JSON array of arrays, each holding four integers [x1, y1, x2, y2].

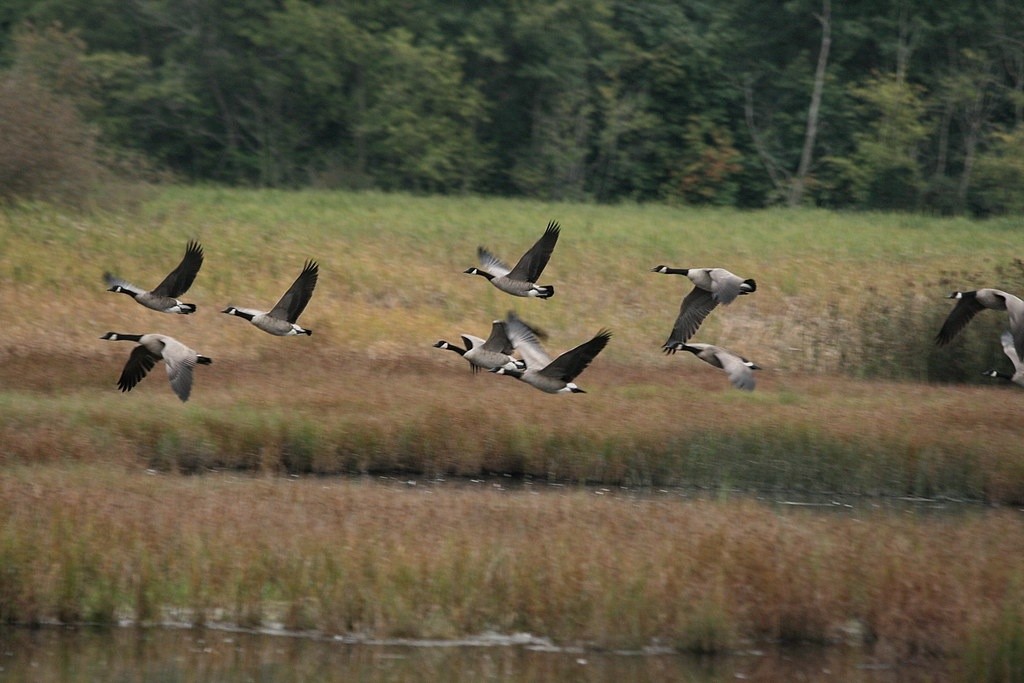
[[432, 310, 613, 395], [102, 239, 204, 315], [462, 220, 561, 300], [650, 265, 757, 355], [220, 258, 320, 336], [979, 330, 1024, 388], [100, 332, 212, 403], [932, 288, 1024, 348], [664, 342, 764, 392]]
